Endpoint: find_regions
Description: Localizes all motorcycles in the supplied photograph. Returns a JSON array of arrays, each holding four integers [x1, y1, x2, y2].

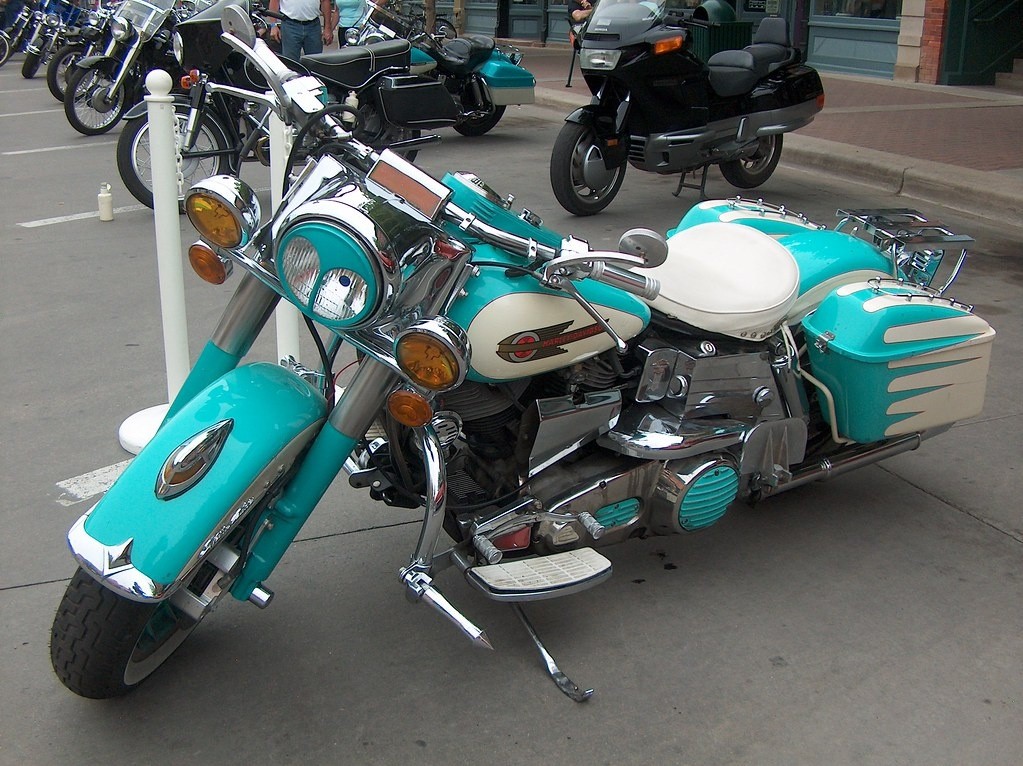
[[0, 0, 539, 219], [49, 2, 998, 709], [546, 1, 828, 218]]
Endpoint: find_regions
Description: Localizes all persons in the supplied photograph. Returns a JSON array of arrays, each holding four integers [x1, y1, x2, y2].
[[568, 0, 593, 49], [269, 0, 333, 59], [324, 0, 386, 48], [1, 0, 25, 52]]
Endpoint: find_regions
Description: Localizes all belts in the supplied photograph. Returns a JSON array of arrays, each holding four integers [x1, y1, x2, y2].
[[286, 16, 318, 25]]
